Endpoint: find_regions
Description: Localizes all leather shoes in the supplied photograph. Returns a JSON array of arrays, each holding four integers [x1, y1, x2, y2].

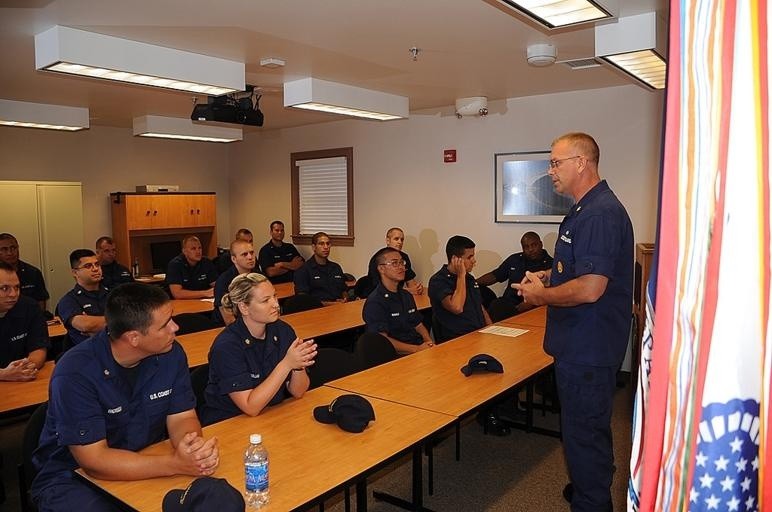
[[476, 412, 511, 435], [499, 406, 527, 420], [564, 483, 574, 504]]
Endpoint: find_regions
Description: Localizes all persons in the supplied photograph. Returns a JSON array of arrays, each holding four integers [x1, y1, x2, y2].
[[0, 228, 314, 511], [511, 132, 634, 512], [476, 231, 554, 323], [293, 232, 349, 306], [258, 221, 305, 282], [368, 227, 424, 295], [428, 235, 529, 437], [364, 247, 436, 355]]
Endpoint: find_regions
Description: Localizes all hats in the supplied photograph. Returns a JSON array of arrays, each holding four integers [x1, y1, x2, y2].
[[461, 354, 503, 376], [162, 478, 245, 512], [314, 395, 375, 432]]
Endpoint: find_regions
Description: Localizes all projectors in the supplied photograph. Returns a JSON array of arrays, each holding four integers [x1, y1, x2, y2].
[[191, 95, 267, 129]]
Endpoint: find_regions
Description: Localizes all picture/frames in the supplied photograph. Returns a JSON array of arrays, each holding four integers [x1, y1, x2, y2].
[[494, 150, 577, 225]]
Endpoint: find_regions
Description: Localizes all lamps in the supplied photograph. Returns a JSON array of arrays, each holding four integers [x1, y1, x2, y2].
[[482, 0, 620, 35], [593, 12, 668, 92], [33, 25, 245, 98], [283, 77, 411, 122], [1, 96, 91, 133], [131, 112, 242, 145]]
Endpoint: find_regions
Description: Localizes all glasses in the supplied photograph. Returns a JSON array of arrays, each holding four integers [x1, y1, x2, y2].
[[379, 260, 407, 267], [549, 156, 581, 168], [76, 263, 99, 270]]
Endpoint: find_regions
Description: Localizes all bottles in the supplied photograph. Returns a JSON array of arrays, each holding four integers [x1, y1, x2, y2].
[[244, 434, 270, 508]]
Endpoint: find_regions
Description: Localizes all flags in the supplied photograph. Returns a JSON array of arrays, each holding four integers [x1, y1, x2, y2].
[[626, 0, 772, 512]]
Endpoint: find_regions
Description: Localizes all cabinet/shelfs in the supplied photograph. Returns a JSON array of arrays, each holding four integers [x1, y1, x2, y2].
[[0, 180, 85, 316], [109, 189, 218, 274]]
